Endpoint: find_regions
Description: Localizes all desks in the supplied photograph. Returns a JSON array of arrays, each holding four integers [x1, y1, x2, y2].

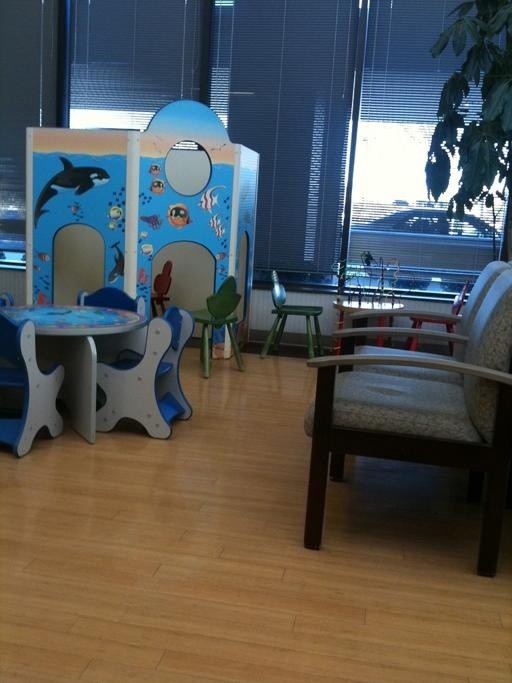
[[0, 306, 147, 444], [333, 301, 405, 355]]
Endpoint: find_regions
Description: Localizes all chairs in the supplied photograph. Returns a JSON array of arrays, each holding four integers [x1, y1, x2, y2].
[[406, 285, 468, 357], [336, 261, 512, 384], [304, 270, 512, 576], [77, 287, 144, 316], [190, 276, 245, 379], [0, 319, 65, 458], [96, 307, 195, 439], [151, 260, 173, 317], [260, 270, 324, 359]]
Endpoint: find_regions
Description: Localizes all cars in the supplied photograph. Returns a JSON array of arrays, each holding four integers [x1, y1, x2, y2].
[[343, 206, 502, 284]]
